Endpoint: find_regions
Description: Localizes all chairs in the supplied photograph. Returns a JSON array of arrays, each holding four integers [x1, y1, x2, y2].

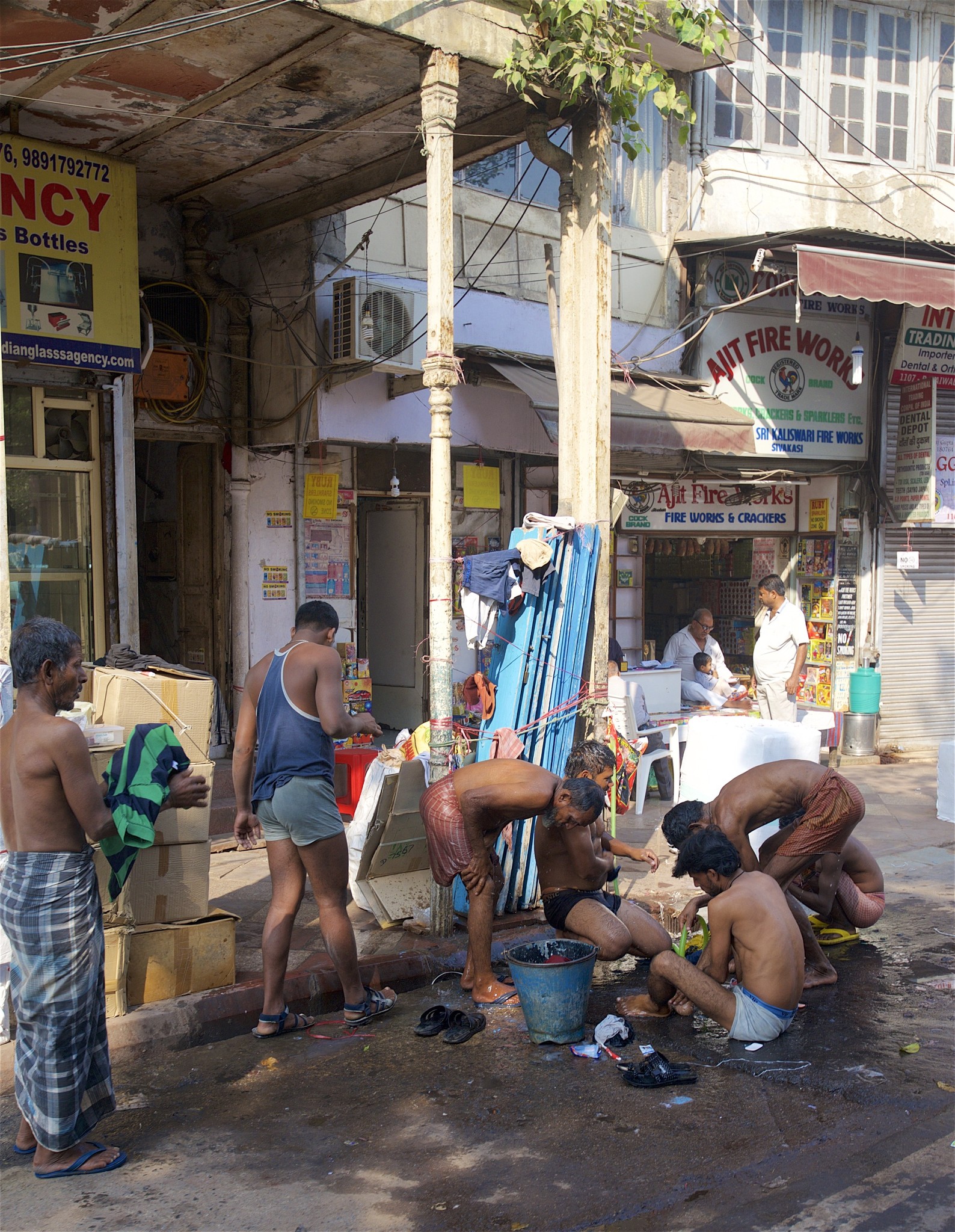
[[609, 695, 680, 817]]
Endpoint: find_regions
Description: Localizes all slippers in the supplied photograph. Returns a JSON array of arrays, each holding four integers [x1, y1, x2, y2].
[[464, 973, 515, 993], [616, 1050, 693, 1074], [413, 1005, 458, 1036], [622, 1056, 699, 1088], [475, 990, 522, 1008], [814, 928, 860, 945], [807, 913, 831, 929], [13, 1143, 37, 1154], [442, 1010, 487, 1042], [35, 1140, 127, 1179]]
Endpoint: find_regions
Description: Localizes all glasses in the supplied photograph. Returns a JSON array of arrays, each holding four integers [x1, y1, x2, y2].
[[695, 619, 714, 631]]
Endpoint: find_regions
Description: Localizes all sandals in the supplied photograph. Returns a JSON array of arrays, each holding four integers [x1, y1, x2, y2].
[[343, 986, 397, 1025], [251, 1003, 316, 1039]]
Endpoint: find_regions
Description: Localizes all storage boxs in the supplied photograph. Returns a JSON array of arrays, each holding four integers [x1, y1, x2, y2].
[[61, 662, 237, 1018]]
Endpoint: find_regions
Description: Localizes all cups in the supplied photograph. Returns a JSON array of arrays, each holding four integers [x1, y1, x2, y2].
[[862, 657, 870, 668]]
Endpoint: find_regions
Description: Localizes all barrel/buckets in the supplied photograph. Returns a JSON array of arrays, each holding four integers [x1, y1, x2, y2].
[[504, 938, 600, 1044], [849, 667, 881, 714], [842, 711, 876, 756]]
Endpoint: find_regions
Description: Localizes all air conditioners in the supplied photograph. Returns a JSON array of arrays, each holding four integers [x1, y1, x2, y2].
[[332, 275, 428, 371]]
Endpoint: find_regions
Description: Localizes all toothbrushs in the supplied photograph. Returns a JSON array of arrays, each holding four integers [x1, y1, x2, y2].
[[599, 1041, 623, 1062]]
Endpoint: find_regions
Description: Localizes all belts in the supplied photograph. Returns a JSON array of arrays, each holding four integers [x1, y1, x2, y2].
[[638, 721, 650, 731]]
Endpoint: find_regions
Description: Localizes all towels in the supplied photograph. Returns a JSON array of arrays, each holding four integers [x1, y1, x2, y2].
[[488, 726, 529, 851], [521, 509, 578, 532]]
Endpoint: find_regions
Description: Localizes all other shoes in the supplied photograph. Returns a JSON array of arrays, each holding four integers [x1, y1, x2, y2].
[[661, 794, 674, 801], [645, 791, 651, 799]]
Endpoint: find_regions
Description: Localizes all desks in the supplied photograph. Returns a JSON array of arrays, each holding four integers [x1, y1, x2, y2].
[[619, 667, 684, 713]]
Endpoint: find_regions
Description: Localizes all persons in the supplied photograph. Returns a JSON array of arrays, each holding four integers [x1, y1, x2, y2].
[[779, 815, 884, 946], [661, 609, 753, 710], [230, 600, 398, 1037], [606, 661, 673, 802], [420, 758, 606, 1011], [753, 573, 809, 722], [2, 615, 210, 1178], [534, 739, 675, 957], [608, 636, 625, 669], [663, 758, 866, 990], [693, 652, 748, 701], [615, 827, 805, 1042]]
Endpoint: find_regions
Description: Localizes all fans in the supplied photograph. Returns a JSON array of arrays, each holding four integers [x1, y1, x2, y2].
[[723, 484, 771, 508]]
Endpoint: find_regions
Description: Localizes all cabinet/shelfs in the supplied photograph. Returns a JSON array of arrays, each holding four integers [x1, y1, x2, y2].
[[796, 573, 833, 710], [609, 532, 644, 667]]
